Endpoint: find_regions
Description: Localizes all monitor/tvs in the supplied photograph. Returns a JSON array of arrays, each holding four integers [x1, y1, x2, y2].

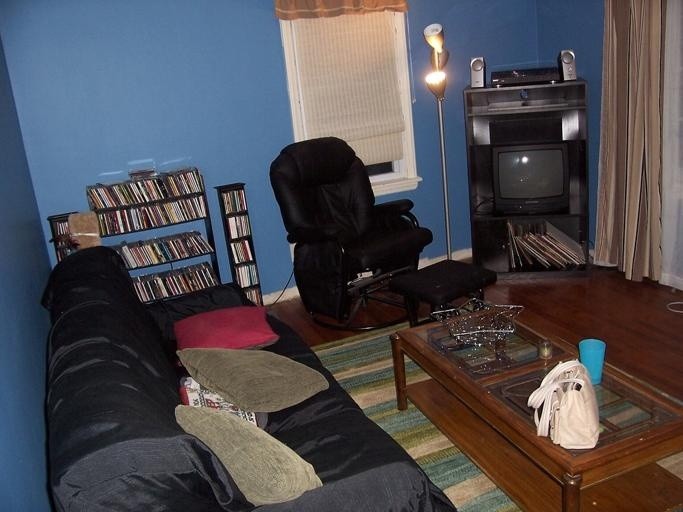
[[490, 142, 570, 216]]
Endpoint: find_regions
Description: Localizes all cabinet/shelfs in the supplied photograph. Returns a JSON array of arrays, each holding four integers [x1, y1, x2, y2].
[[462, 79, 589, 277], [213, 181, 266, 305], [46, 212, 78, 263], [87, 176, 220, 303]]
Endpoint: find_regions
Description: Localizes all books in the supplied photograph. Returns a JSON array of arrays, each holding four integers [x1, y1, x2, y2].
[[55, 165, 262, 306], [505, 217, 584, 271]]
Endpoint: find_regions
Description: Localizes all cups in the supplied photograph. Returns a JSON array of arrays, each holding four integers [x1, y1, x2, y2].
[[577, 338, 606, 386]]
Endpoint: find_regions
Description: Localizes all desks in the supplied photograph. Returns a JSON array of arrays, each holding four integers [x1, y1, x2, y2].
[[389, 304, 681, 511]]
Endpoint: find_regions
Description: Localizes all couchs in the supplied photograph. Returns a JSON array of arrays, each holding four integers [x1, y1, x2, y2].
[[39, 245, 455, 511]]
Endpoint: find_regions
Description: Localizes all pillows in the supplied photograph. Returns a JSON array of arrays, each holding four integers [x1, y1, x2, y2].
[[170, 304, 282, 351], [175, 346, 331, 415], [172, 401, 325, 508]]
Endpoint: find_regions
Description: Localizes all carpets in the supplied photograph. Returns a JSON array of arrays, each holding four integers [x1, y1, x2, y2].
[[309, 309, 683, 511]]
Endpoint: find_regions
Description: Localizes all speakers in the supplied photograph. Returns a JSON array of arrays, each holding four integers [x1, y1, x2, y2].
[[557, 49, 577, 81], [470, 56, 486, 88]]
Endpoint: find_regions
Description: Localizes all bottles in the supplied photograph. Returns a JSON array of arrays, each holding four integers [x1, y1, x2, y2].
[[538, 340, 552, 360]]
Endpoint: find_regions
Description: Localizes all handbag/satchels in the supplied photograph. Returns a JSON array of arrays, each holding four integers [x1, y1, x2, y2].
[[528, 359, 601, 451]]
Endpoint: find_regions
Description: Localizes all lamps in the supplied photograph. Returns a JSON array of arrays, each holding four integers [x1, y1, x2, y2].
[[422, 21, 456, 259]]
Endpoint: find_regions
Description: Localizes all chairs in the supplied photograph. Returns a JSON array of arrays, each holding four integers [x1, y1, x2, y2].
[[267, 135, 436, 334]]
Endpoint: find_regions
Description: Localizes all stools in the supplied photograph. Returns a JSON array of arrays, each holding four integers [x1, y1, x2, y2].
[[390, 256, 498, 328]]
